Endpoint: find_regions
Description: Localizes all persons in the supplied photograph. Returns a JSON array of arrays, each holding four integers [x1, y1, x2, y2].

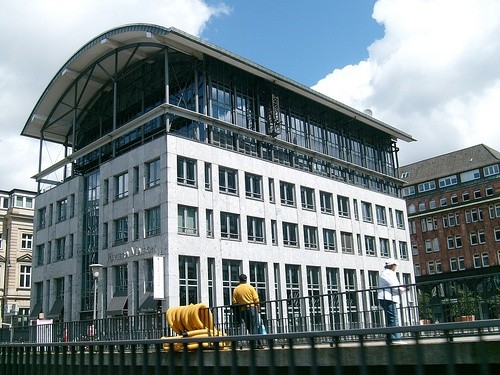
[[231, 273, 270, 350], [376, 255, 409, 344]]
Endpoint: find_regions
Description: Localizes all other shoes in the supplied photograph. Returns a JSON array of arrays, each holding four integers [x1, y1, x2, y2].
[[390, 340, 406, 345], [256, 346, 264, 349]]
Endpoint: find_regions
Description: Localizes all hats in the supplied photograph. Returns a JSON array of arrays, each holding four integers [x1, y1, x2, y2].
[[385, 258, 397, 266]]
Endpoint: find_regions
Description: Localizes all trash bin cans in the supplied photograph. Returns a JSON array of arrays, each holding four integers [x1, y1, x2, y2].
[[32, 319, 53, 351]]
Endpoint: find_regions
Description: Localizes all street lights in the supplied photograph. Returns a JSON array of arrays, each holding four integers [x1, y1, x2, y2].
[[89, 263, 103, 351]]
